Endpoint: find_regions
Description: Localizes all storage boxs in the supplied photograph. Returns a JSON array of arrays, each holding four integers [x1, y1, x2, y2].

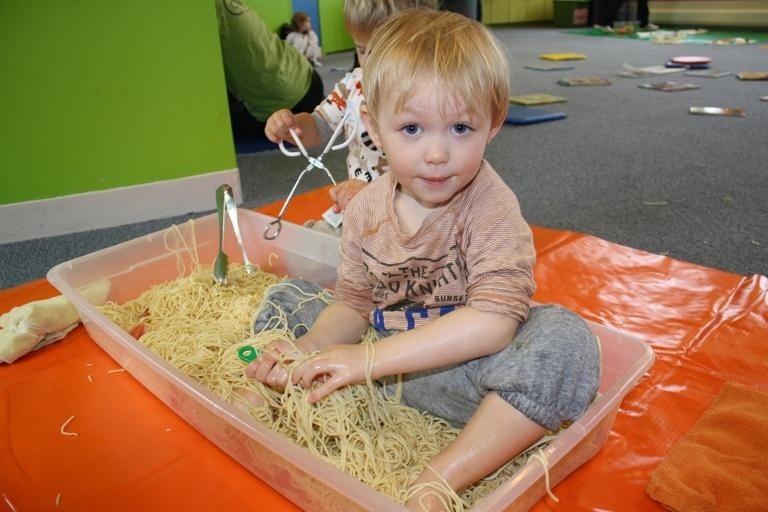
[[45, 203, 656, 512]]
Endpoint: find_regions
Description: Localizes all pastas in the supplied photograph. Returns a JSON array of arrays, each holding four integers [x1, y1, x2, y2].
[[100, 256, 561, 512]]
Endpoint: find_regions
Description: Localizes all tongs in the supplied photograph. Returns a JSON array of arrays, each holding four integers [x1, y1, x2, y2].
[[213, 185, 253, 283], [262, 109, 358, 240]]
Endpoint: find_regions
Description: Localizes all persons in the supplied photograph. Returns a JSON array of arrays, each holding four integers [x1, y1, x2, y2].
[[284, 10, 324, 68], [228, 4, 604, 512], [263, 0, 442, 238], [214, 0, 325, 157]]
